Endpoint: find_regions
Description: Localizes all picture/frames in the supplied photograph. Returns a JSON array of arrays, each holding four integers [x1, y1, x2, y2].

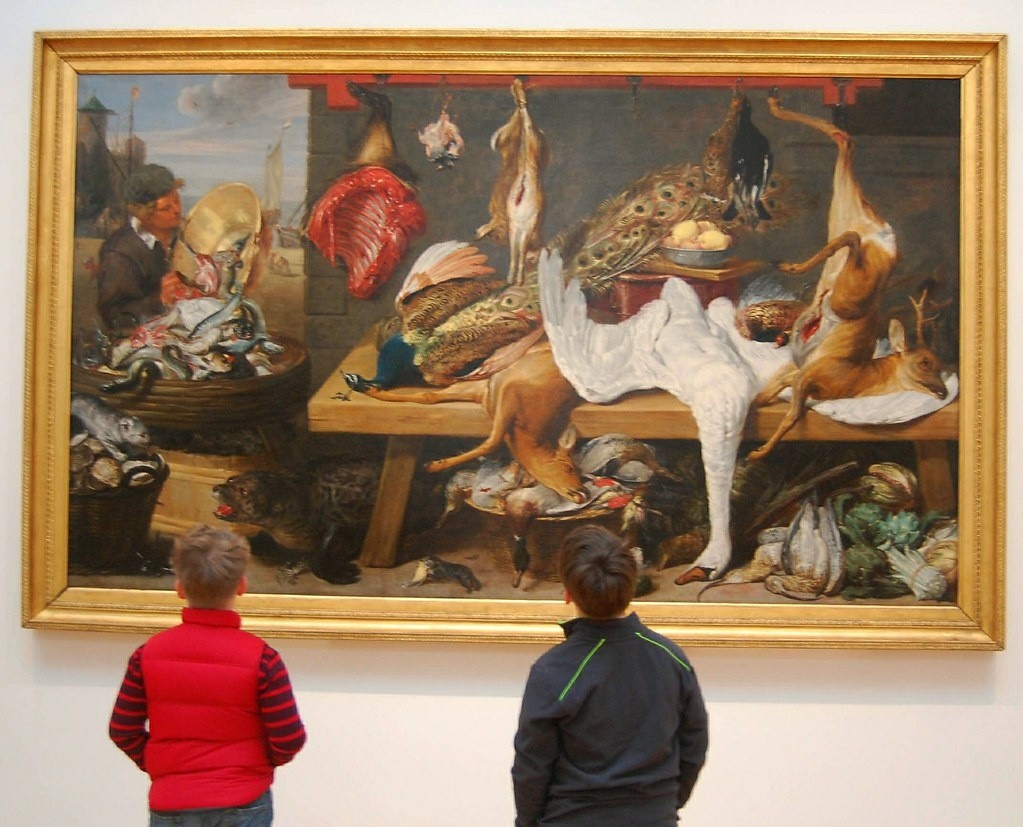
[[21, 27, 1018, 649]]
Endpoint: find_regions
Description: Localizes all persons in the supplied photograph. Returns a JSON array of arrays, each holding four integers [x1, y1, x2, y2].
[[511, 524, 708, 827], [109, 523, 306, 827]]
